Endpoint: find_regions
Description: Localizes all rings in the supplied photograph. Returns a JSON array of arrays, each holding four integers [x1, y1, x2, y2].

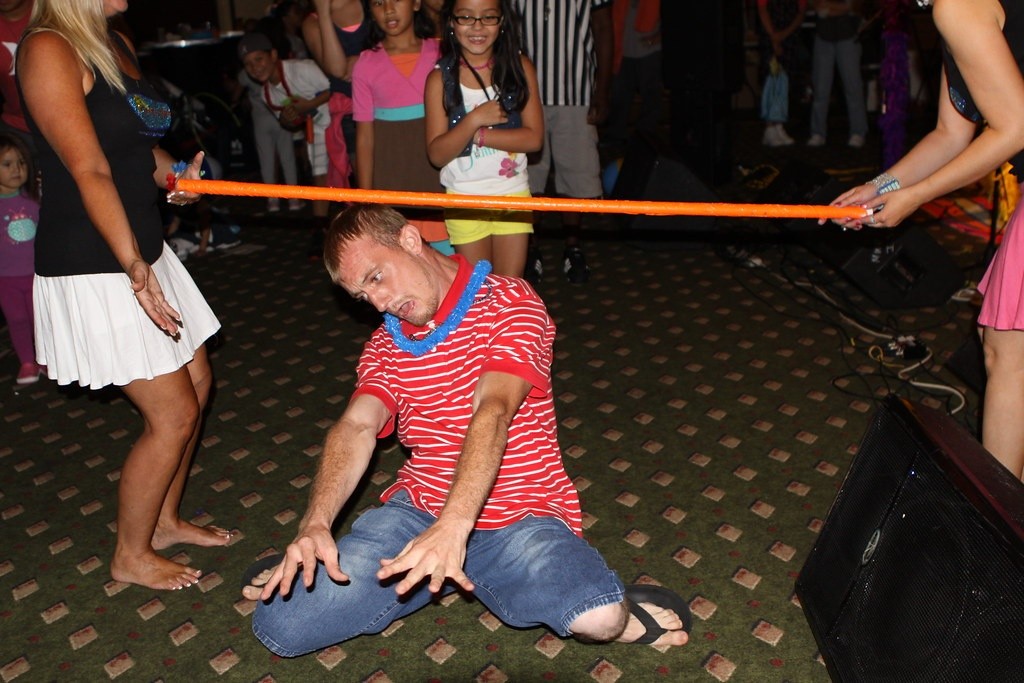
[[871, 216, 878, 224]]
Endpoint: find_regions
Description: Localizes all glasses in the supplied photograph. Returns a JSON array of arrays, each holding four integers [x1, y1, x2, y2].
[[449, 11, 504, 26]]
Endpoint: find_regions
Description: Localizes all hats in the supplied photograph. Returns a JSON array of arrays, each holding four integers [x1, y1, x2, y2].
[[237, 32, 273, 57]]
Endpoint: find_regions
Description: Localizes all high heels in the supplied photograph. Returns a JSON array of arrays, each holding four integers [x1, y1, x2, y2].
[[762, 122, 796, 146]]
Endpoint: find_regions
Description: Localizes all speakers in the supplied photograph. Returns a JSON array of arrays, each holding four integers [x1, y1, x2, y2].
[[784, 181, 966, 307], [611, 129, 720, 231], [793, 391, 1024, 683]]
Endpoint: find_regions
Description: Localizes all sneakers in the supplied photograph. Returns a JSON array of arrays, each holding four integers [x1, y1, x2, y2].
[[518, 247, 543, 295], [562, 243, 590, 283]]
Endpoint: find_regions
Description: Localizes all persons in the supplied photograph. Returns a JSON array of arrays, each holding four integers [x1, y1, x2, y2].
[[817, 0, 1024, 483], [352, 0, 615, 289], [15, 0, 234, 591], [240, 203, 693, 658], [222, 0, 385, 257], [758, 0, 869, 148], [0, 0, 48, 384]]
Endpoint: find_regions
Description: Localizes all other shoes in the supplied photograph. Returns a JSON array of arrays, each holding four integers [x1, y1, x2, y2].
[[290, 200, 306, 210], [16, 358, 50, 384], [849, 133, 866, 148], [806, 133, 826, 146], [263, 198, 282, 212]]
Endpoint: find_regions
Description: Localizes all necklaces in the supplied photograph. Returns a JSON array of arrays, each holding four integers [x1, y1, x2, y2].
[[459, 57, 495, 70], [263, 60, 304, 125]]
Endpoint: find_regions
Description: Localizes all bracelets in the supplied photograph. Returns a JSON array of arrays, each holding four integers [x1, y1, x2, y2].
[[478, 127, 485, 147], [865, 172, 901, 211], [164, 160, 205, 192]]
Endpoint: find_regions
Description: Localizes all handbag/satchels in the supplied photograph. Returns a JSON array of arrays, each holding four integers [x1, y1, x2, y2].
[[759, 48, 791, 122]]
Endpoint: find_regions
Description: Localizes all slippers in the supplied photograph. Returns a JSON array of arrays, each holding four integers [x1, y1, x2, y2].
[[623, 584, 692, 646]]
